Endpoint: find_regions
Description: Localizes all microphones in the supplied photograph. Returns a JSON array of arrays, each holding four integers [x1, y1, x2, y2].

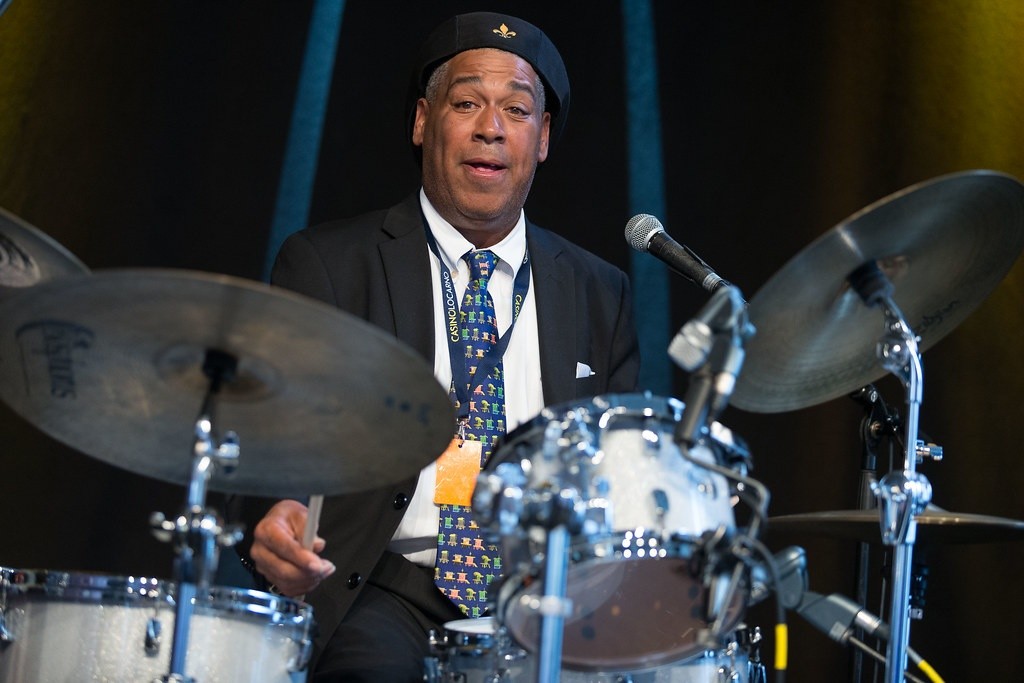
[[625, 214, 728, 292], [668, 285, 746, 372]]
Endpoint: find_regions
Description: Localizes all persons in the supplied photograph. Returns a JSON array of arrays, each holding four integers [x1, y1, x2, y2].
[[246, 11, 640, 683]]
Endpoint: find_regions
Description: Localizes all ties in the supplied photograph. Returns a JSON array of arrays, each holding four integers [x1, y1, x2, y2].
[[433, 251, 508, 621]]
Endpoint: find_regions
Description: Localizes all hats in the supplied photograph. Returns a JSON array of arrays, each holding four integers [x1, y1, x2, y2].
[[404, 11, 570, 172]]
